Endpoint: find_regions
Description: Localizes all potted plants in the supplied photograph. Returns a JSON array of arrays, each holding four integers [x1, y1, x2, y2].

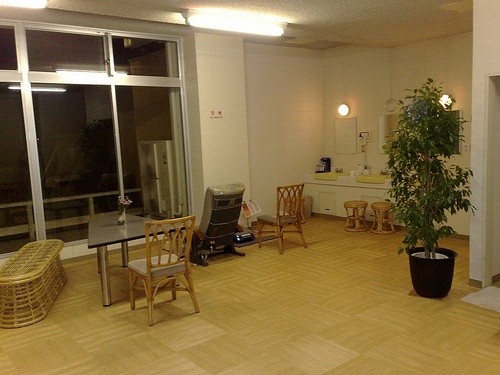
[[378, 76, 479, 300]]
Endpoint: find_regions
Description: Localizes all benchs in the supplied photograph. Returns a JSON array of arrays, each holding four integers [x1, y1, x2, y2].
[[0, 239, 68, 329]]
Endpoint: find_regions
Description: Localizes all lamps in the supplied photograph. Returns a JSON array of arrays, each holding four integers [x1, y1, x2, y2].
[[439, 93, 452, 109], [8, 83, 67, 95], [180, 9, 288, 37], [338, 102, 350, 117]]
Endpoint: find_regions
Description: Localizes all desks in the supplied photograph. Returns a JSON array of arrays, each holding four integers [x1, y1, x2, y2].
[[85, 207, 189, 307]]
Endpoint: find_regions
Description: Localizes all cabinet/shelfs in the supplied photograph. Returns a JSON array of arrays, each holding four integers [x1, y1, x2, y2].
[[304, 180, 469, 236]]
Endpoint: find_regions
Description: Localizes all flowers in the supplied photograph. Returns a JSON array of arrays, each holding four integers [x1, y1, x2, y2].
[[117, 194, 133, 206]]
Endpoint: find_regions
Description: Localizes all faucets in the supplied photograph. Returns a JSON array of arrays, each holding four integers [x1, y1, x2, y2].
[[335, 168, 343, 173], [379, 169, 388, 175]]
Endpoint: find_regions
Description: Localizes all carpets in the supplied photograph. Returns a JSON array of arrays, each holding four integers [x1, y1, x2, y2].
[[460, 284, 500, 313]]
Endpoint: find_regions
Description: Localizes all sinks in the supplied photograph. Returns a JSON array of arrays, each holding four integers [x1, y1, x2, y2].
[[315, 172, 348, 181], [356, 175, 386, 184]]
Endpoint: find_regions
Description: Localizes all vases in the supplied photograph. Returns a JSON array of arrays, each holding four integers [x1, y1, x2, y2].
[[118, 207, 126, 225]]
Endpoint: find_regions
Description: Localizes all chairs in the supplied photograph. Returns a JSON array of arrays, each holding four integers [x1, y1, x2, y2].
[[181, 182, 247, 268], [127, 213, 202, 328], [256, 183, 306, 256]]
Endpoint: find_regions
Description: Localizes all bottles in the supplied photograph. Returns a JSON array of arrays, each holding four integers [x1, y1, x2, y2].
[[340, 168, 343, 176], [335, 168, 340, 176]]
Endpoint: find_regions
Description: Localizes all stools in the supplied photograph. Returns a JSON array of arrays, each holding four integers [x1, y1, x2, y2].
[[370, 199, 395, 235], [288, 196, 313, 218], [343, 200, 369, 232]]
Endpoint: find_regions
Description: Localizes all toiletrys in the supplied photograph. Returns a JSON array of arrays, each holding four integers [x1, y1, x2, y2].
[[315, 161, 326, 171], [362, 164, 370, 176]]
